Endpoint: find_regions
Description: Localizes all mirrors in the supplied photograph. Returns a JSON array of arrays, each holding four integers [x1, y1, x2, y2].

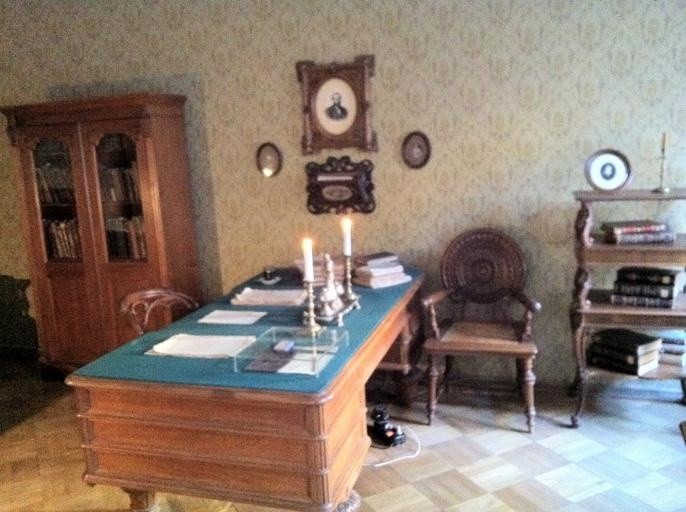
[[300, 154, 378, 220]]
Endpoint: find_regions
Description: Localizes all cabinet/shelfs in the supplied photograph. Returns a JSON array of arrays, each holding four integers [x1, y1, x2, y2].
[[0, 86, 203, 386], [557, 182, 686, 449]]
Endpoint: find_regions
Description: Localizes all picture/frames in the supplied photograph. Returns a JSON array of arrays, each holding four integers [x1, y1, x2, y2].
[[583, 148, 632, 194], [292, 51, 381, 159], [400, 131, 431, 168], [255, 141, 282, 178]]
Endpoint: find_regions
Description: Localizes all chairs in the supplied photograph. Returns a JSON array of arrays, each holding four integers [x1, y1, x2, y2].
[[413, 223, 544, 437], [116, 284, 203, 342], [0, 272, 42, 389]]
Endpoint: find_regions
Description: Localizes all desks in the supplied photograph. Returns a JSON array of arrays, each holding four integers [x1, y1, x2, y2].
[[58, 259, 432, 512]]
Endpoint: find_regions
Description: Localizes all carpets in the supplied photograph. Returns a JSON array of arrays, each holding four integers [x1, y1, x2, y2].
[[0, 363, 74, 436]]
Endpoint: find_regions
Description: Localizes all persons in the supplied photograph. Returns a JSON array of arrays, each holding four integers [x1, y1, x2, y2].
[[324, 91, 347, 122]]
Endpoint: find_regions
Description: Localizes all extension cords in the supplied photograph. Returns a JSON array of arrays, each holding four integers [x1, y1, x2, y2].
[[367, 424, 407, 447]]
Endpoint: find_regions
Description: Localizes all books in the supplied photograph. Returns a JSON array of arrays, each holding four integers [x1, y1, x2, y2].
[[583, 215, 686, 377], [293, 251, 412, 290], [228, 284, 309, 307], [35, 160, 146, 261]]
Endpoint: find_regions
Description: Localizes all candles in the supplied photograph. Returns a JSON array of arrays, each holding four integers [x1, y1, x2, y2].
[[661, 132, 668, 150], [300, 240, 315, 281], [338, 218, 354, 256]]
